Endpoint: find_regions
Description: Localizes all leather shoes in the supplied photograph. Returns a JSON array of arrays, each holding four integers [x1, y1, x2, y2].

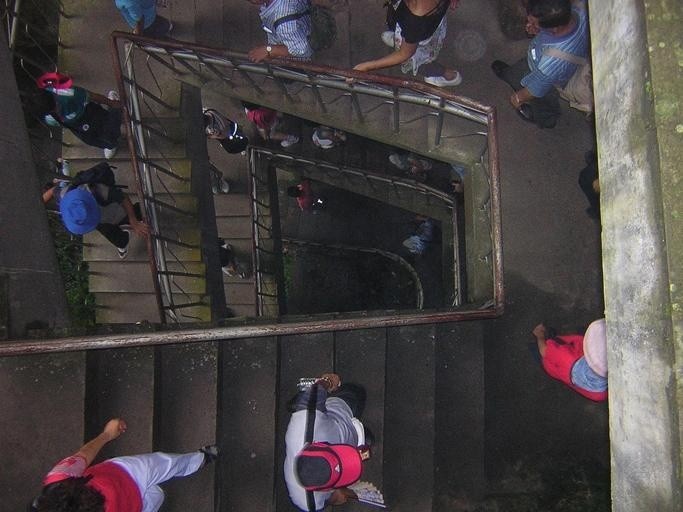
[[518, 103, 555, 128], [491, 60, 511, 82]]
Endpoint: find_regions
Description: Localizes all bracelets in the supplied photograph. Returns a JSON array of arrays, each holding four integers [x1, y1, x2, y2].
[[313, 376, 332, 390]]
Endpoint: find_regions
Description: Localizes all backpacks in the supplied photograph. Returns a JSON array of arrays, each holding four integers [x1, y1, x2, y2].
[[274, 4, 337, 51], [543, 48, 594, 119], [66, 162, 117, 206]]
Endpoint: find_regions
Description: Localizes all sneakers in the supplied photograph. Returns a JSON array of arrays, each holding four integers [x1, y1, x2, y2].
[[380, 31, 396, 48], [117, 230, 131, 259], [281, 137, 300, 148], [424, 69, 462, 87], [199, 445, 223, 462], [104, 146, 117, 160]]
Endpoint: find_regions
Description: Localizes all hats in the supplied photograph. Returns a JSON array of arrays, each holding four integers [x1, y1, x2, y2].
[[583, 318, 608, 378], [295, 441, 363, 491], [60, 189, 101, 235]]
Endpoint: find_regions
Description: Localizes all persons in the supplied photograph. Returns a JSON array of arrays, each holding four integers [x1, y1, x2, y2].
[[25, 418, 221, 511], [219, 237, 250, 279], [346, 1, 462, 87], [530, 318, 606, 403], [42, 179, 152, 260], [491, 1, 600, 215], [29, 86, 123, 160], [386, 148, 464, 258], [203, 99, 347, 215], [283, 372, 373, 511], [114, 1, 174, 41], [248, 1, 314, 74]]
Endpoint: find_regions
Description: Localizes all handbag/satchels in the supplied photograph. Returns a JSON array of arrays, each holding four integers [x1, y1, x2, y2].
[[74, 104, 97, 136], [221, 135, 248, 154]]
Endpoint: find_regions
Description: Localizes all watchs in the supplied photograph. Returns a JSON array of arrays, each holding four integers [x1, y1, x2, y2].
[[265, 45, 272, 55]]
